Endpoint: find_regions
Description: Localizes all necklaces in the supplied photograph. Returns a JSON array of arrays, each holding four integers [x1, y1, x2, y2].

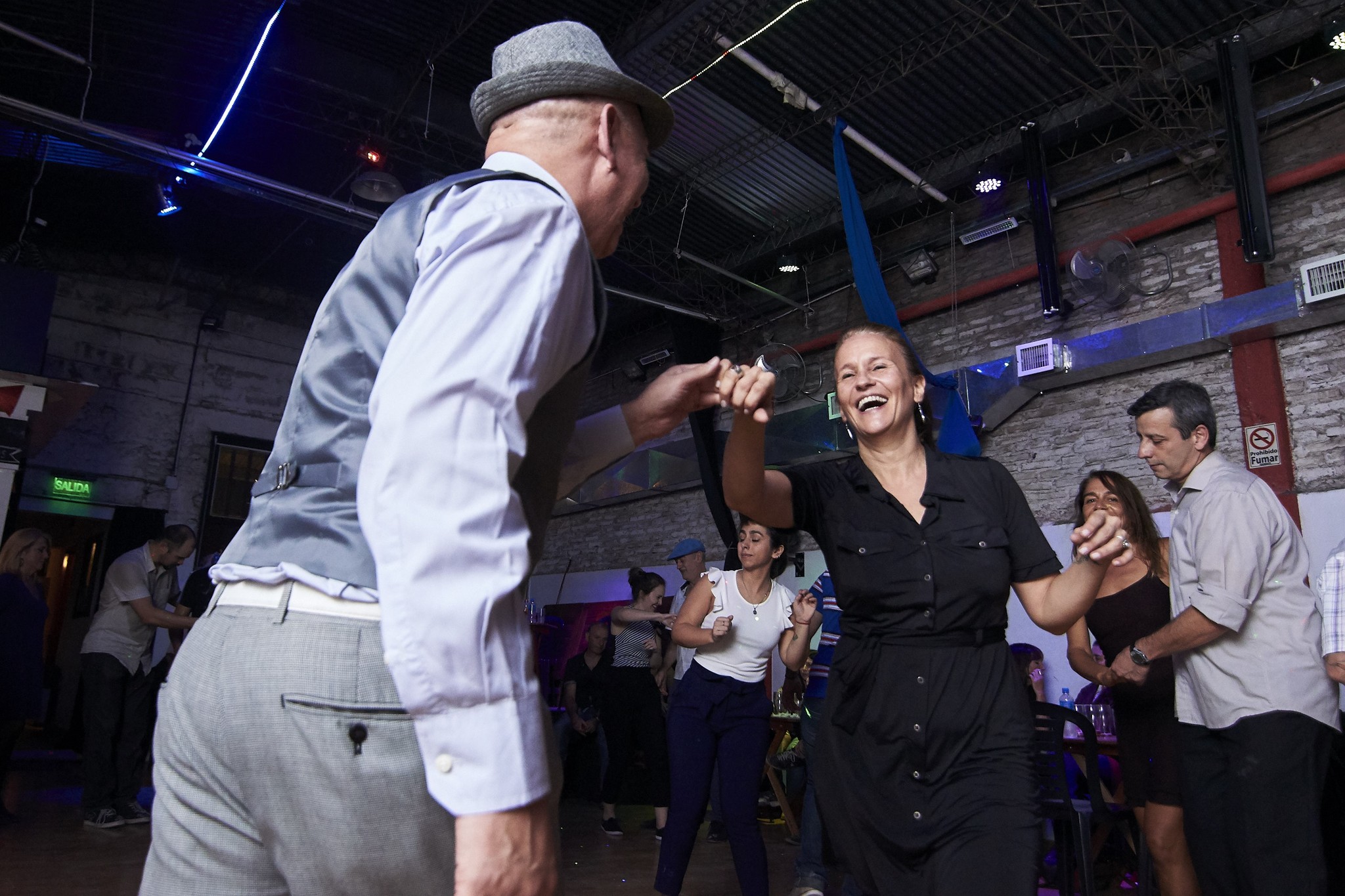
[[741, 569, 770, 616]]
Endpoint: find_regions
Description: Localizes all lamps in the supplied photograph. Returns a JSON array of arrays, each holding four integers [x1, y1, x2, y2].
[[959, 217, 1018, 246], [897, 245, 937, 290], [972, 158, 1004, 195], [776, 254, 799, 274], [1326, 20, 1345, 52], [351, 171, 405, 204], [153, 176, 182, 217]]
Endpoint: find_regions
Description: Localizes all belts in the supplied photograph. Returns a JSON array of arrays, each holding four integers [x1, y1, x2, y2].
[[216, 579, 384, 620]]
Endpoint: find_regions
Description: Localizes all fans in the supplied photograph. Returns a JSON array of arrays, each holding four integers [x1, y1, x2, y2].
[[749, 342, 826, 401], [1064, 230, 1174, 314]]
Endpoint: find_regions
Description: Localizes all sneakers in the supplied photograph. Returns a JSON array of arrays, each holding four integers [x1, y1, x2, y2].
[[758, 792, 780, 807], [788, 887, 822, 896], [766, 748, 805, 770], [601, 818, 623, 835], [656, 827, 664, 839], [114, 802, 151, 824], [84, 807, 125, 828]]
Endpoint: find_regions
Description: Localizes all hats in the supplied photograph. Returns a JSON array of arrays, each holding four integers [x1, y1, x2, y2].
[[470, 21, 674, 151], [667, 539, 706, 561]]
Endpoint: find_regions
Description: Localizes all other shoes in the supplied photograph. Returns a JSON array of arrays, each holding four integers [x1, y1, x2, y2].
[[706, 833, 727, 842], [0, 800, 20, 828]]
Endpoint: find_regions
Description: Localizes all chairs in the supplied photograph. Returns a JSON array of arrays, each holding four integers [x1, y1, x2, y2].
[[1032, 700, 1138, 895]]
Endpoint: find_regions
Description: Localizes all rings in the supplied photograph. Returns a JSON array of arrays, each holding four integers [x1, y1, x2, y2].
[[729, 364, 744, 379], [1117, 535, 1128, 549], [1039, 670, 1043, 675]]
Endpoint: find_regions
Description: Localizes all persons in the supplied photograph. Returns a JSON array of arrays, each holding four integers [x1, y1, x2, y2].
[[0, 528, 52, 823], [75, 326, 1345, 896], [137, 19, 674, 896]]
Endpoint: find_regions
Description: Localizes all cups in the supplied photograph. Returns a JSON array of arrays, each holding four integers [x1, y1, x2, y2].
[[794, 692, 804, 707], [537, 608, 546, 624], [773, 692, 785, 714], [1074, 704, 1116, 736]]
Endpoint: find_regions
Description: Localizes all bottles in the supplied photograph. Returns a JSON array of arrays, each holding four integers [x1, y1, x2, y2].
[[1059, 687, 1076, 711], [526, 598, 536, 623]]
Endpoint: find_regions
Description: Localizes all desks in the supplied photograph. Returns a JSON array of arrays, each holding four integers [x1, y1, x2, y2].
[[1057, 730, 1122, 758], [758, 708, 803, 844]]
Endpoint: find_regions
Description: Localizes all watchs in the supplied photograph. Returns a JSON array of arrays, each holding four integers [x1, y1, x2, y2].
[[1129, 639, 1154, 667]]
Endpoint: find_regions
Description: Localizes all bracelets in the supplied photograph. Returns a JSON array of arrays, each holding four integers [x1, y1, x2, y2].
[[794, 618, 810, 625]]
